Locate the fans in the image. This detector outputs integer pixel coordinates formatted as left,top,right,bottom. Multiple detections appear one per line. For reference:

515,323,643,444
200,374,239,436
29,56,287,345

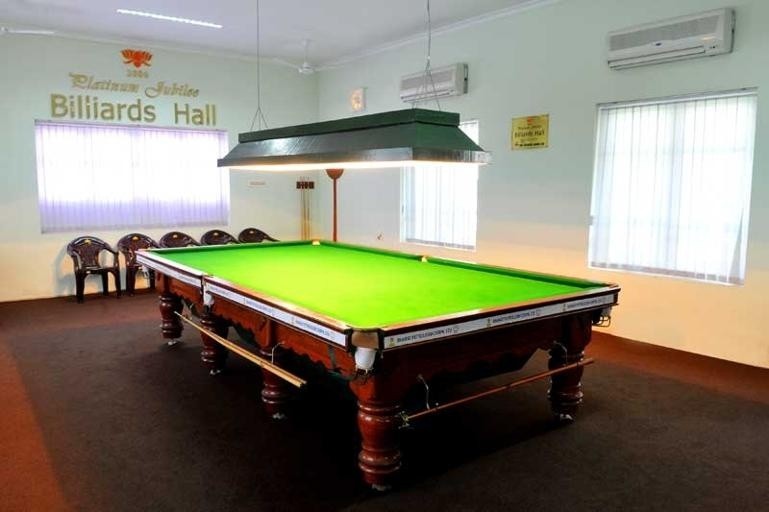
272,56,346,80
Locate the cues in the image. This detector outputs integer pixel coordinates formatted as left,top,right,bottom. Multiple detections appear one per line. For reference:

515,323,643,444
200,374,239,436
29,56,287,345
300,176,310,239
397,358,595,429
174,311,303,388
204,329,307,386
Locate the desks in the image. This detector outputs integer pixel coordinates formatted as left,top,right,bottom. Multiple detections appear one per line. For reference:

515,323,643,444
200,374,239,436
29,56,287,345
134,239,621,490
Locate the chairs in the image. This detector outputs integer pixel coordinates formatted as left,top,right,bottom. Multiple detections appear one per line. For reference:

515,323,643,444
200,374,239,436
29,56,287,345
238,228,281,243
159,231,204,248
201,229,241,246
67,236,121,304
118,232,163,296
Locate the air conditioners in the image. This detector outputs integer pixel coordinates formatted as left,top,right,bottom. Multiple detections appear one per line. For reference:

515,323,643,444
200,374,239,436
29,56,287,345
607,7,736,71
399,63,468,102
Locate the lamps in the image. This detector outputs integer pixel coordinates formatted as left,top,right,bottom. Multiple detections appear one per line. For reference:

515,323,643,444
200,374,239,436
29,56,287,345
217,108,493,170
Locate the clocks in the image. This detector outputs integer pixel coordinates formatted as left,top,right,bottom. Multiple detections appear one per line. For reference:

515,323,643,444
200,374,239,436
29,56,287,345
350,87,365,111
